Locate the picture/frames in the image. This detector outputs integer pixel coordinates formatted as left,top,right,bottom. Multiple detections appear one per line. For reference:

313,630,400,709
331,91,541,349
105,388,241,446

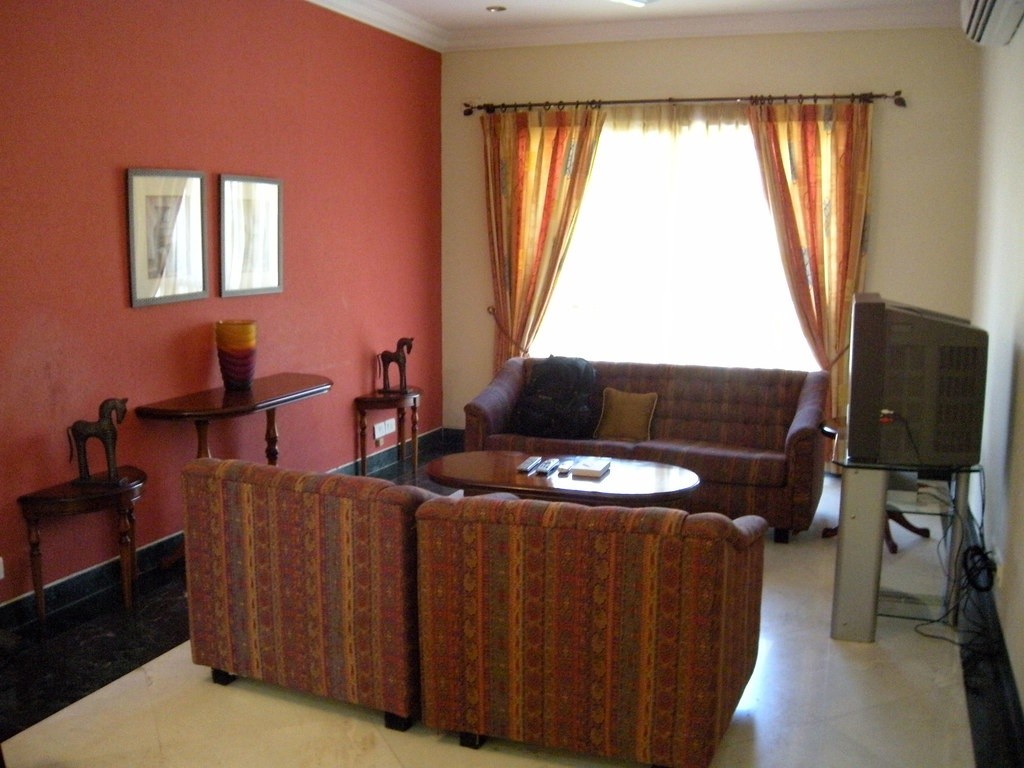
219,173,283,299
126,167,210,308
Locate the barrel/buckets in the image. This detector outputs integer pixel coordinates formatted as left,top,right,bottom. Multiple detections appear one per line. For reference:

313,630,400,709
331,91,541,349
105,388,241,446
215,319,256,393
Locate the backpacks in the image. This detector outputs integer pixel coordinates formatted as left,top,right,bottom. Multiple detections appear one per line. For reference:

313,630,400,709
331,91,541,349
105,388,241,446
513,356,598,440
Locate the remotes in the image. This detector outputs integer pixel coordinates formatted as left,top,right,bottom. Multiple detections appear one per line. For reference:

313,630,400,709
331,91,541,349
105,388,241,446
537,458,560,474
517,457,542,472
559,461,573,473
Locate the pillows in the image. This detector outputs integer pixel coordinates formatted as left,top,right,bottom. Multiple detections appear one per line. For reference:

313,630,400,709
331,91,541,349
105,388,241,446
592,388,658,443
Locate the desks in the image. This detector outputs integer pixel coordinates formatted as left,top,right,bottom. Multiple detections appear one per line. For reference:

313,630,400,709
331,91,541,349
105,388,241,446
425,450,700,511
135,373,333,468
830,430,983,645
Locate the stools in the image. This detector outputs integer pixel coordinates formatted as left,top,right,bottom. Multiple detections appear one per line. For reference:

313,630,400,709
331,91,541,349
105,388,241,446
820,416,930,554
16,465,148,628
353,385,425,478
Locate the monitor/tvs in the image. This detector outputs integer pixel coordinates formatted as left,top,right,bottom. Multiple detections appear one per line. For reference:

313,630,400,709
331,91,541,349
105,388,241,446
847,291,989,467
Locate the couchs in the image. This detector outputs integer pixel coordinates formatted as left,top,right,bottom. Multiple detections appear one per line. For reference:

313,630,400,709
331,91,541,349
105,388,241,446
416,493,768,768
181,457,464,732
464,354,830,545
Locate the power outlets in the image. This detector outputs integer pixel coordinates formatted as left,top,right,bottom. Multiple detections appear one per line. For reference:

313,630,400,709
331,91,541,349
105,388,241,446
992,547,1004,587
374,421,385,439
384,418,396,434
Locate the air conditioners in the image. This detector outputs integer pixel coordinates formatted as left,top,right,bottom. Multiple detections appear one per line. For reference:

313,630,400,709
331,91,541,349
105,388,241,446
960,0,1024,47
